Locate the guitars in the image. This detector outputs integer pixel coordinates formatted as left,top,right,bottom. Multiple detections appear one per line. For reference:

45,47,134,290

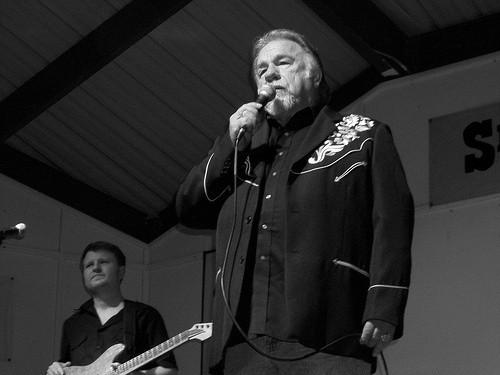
45,321,214,375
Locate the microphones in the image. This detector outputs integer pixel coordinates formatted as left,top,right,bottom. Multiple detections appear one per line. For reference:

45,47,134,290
0,223,26,241
237,84,276,141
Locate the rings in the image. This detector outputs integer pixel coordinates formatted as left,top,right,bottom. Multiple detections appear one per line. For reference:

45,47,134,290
241,109,247,115
381,333,391,343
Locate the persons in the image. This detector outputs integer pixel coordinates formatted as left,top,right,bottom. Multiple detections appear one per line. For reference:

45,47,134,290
176,27,413,375
45,240,179,375
